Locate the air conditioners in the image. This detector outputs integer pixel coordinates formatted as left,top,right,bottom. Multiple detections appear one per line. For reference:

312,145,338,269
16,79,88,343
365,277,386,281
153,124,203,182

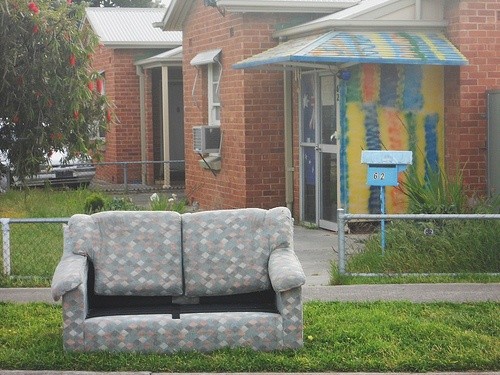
193,125,220,152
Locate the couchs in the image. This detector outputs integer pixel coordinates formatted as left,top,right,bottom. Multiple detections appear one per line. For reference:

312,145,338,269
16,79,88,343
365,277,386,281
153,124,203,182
51,207,306,354
